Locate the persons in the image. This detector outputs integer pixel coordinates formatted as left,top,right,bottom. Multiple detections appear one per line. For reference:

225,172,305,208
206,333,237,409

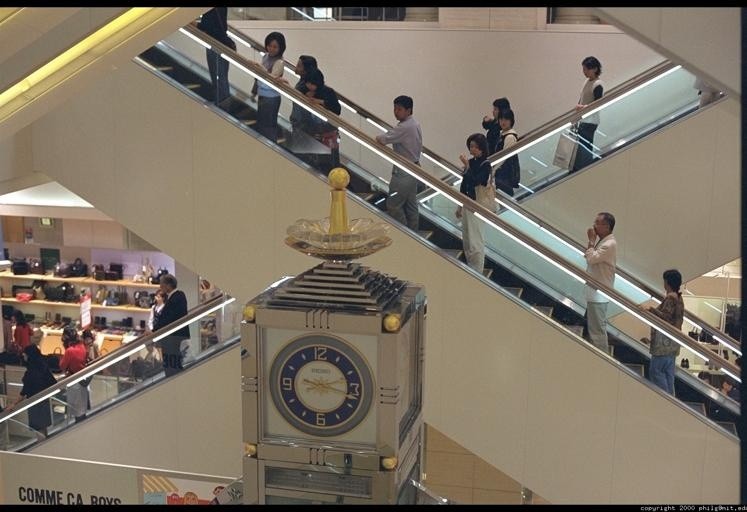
56,327,91,423
248,31,288,140
80,328,103,362
373,94,423,232
303,67,341,178
582,212,618,357
484,97,510,154
568,56,605,172
189,5,236,104
694,76,721,109
495,110,520,209
697,371,742,421
279,54,318,167
10,309,42,357
638,267,686,399
144,343,162,363
455,131,494,277
153,273,191,378
147,287,167,331
4,343,57,443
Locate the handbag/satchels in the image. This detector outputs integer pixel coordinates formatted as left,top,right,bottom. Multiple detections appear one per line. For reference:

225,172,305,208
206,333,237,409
553,133,579,173
224,35,237,50
417,163,426,195
476,182,500,212
322,129,339,150
0,258,217,419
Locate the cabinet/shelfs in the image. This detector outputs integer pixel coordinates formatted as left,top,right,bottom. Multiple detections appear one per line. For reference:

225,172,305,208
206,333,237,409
1,272,161,342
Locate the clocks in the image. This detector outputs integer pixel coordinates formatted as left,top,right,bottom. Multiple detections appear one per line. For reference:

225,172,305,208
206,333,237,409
268,334,373,435
